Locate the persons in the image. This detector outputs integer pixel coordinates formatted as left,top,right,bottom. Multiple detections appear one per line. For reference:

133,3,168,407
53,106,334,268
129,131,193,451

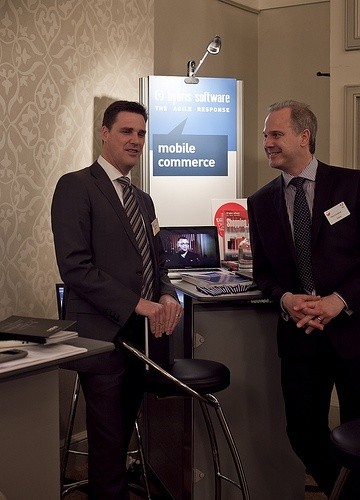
247,100,360,500
50,101,184,500
173,237,200,264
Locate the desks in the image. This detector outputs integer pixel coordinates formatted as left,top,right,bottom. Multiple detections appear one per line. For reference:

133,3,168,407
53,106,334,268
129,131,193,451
0,338,114,500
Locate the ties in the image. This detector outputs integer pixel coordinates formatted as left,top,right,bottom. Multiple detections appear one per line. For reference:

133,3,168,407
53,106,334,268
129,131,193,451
290,177,316,294
117,175,154,300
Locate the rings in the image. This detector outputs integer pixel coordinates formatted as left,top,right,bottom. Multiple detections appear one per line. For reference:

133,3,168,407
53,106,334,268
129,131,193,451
317,317,321,321
176,314,179,317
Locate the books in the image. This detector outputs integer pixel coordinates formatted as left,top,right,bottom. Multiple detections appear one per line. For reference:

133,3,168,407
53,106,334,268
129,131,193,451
0,315,78,344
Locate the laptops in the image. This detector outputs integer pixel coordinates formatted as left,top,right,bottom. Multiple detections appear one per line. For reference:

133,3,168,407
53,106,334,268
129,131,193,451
158,225,234,279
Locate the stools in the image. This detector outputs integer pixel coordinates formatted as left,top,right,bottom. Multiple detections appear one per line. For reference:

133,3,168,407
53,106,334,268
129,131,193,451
59,367,151,500
124,318,252,500
328,419,360,500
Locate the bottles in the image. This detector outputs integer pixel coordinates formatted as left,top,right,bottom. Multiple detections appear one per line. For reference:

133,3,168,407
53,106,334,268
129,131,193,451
238,226,253,272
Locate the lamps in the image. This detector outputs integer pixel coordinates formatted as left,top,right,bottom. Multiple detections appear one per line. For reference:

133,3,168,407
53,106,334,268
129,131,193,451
186,35,221,83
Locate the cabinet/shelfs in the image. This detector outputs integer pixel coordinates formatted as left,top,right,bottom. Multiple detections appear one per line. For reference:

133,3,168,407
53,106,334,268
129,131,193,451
170,274,307,500
